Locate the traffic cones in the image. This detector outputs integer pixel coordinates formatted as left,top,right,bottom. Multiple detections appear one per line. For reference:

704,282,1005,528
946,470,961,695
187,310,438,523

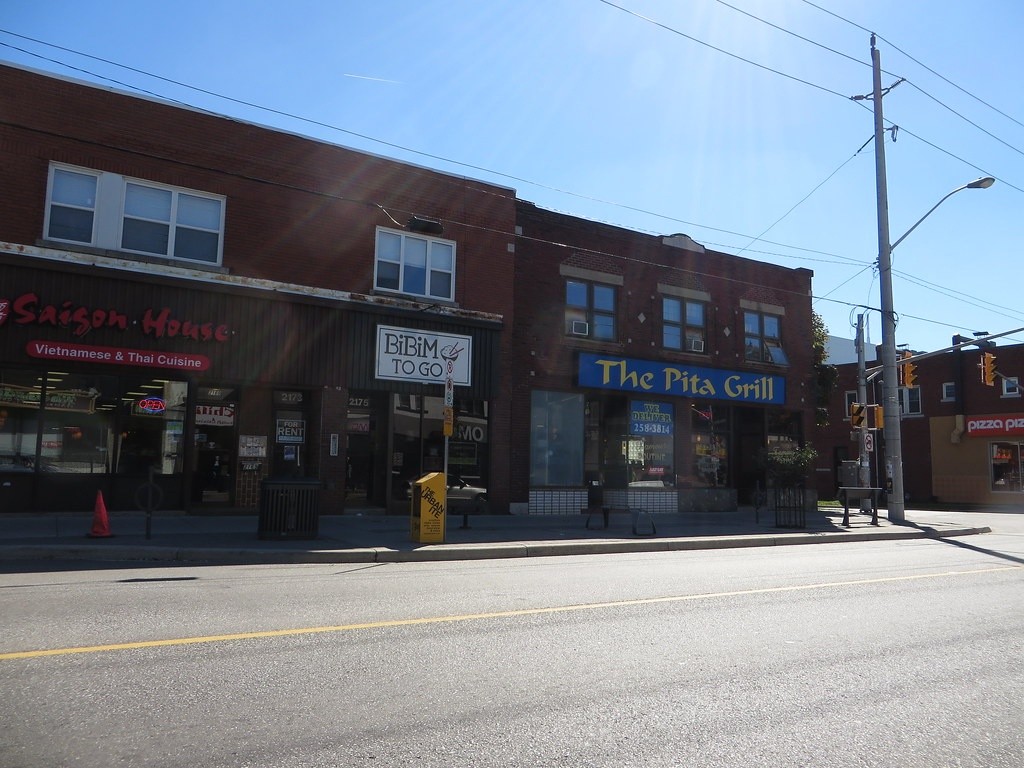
84,489,116,539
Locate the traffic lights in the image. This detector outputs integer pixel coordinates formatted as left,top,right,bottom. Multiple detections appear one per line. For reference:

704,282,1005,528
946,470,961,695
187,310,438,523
850,401,866,428
867,405,885,430
984,353,997,387
905,350,918,388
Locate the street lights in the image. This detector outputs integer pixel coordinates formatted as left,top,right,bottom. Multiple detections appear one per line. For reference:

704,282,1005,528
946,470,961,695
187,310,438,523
873,173,995,526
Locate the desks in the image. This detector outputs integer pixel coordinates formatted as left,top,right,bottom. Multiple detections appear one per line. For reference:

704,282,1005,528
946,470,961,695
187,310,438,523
584,504,658,538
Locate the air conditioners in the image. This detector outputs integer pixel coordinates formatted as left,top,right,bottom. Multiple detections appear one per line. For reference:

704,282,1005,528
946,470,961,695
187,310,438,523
570,320,590,337
686,339,705,353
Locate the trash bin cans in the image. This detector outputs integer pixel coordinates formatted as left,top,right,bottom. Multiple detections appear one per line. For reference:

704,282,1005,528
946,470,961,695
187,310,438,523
257,470,323,539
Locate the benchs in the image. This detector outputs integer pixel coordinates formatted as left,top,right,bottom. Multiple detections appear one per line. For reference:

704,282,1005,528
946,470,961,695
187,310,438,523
838,486,882,529
